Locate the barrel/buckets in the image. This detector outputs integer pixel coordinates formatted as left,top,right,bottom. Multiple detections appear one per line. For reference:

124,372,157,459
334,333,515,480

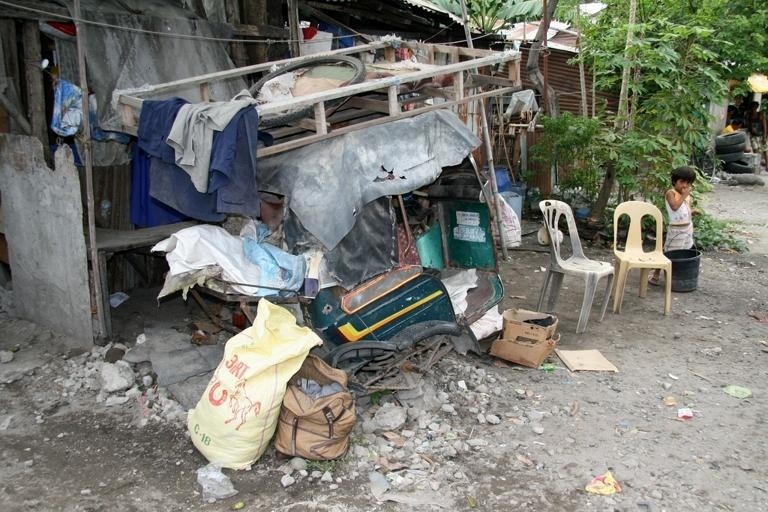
664,232,702,294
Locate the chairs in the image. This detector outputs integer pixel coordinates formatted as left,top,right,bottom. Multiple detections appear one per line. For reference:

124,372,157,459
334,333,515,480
537,199,615,335
611,200,673,317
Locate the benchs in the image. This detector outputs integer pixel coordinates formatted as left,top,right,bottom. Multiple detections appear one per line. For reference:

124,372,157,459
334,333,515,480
91,220,224,340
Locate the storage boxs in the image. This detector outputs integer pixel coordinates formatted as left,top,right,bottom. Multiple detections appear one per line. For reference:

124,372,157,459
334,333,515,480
490,308,562,369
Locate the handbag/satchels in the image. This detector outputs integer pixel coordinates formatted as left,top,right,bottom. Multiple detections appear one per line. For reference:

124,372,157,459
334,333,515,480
272,353,357,462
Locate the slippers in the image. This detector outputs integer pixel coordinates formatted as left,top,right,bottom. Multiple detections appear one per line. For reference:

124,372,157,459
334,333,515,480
649,274,661,285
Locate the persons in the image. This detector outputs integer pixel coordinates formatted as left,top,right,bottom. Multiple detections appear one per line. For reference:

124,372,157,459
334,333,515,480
721,94,768,171
649,165,704,286
